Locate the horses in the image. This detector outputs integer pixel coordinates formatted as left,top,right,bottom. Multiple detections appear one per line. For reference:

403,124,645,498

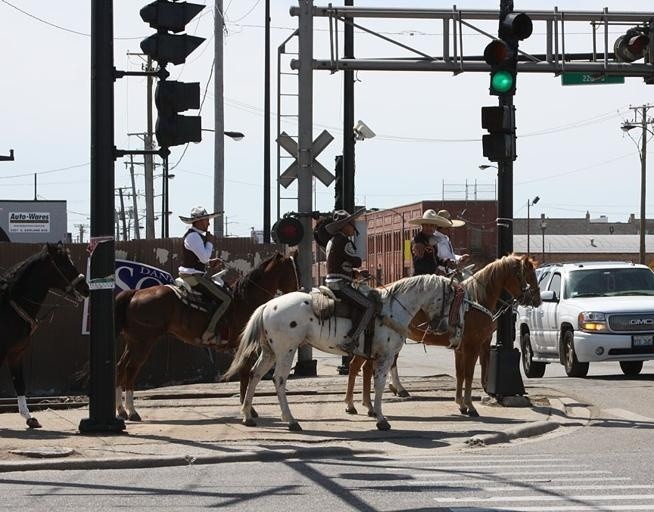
221,273,466,432
1,238,93,430
345,252,542,418
114,249,298,424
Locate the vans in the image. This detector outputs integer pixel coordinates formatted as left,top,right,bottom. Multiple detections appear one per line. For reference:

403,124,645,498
514,261,654,380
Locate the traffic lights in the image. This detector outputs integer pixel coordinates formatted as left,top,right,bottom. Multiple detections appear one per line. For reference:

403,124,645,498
140,0,209,67
481,13,533,98
478,105,518,161
149,78,204,148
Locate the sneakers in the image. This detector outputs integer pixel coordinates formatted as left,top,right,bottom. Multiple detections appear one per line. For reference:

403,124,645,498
432,325,448,336
342,337,360,355
204,335,228,346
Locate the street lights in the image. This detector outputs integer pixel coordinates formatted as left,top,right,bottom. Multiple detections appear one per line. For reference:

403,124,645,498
109,112,246,241
371,202,407,279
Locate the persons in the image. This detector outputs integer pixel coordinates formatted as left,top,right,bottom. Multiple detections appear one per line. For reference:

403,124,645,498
407,206,465,335
433,209,469,277
321,208,375,355
176,206,232,345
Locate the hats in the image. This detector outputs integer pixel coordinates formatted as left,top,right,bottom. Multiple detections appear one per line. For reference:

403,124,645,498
180,206,225,223
409,209,465,228
325,207,366,235
